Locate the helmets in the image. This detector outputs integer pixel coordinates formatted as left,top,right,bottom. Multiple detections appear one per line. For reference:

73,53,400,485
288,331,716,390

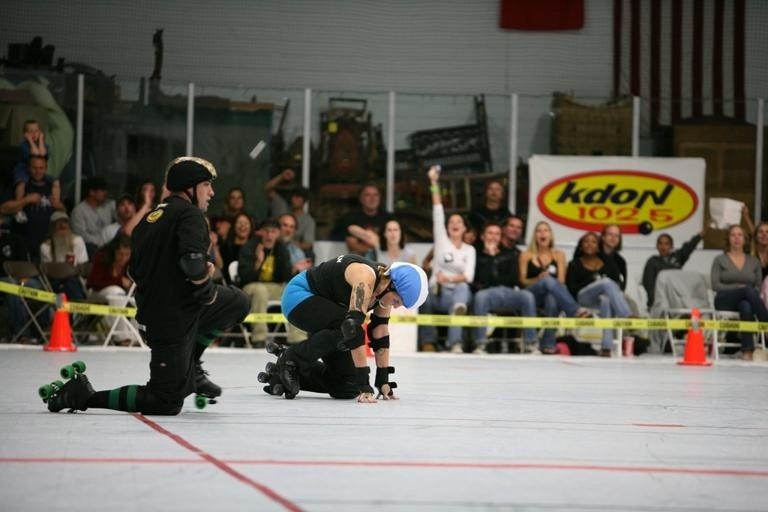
164,156,215,191
387,261,429,309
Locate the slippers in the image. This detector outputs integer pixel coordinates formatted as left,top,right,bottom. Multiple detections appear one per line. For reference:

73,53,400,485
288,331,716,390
540,344,561,354
571,310,594,319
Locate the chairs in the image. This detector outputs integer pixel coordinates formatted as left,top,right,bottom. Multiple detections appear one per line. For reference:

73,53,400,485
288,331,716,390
0,260,79,345
102,282,144,347
711,290,764,358
632,272,651,339
228,261,292,349
658,270,718,359
37,261,108,345
567,306,622,357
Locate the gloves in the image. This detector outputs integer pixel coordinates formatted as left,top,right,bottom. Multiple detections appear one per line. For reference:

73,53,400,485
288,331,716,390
374,367,399,397
352,367,375,394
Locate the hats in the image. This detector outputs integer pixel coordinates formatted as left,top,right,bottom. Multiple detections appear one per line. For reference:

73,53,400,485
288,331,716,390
116,192,135,204
86,176,107,188
49,209,69,223
258,216,283,230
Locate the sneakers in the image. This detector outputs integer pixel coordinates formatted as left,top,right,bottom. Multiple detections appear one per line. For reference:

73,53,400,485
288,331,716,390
266,343,312,400
451,341,464,354
628,316,648,333
258,362,284,397
14,208,29,225
597,346,614,355
190,362,221,410
452,301,468,316
525,343,544,356
38,360,96,415
472,344,487,353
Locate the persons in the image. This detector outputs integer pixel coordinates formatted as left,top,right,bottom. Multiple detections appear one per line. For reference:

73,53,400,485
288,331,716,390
518,222,600,356
599,225,652,356
113,190,158,238
712,225,768,362
278,213,308,273
136,180,159,215
420,165,478,354
212,217,235,247
239,217,292,283
643,222,717,342
346,186,395,252
209,215,256,287
567,233,649,358
263,168,316,250
744,202,768,314
86,239,134,346
472,224,541,355
464,181,515,243
0,154,67,261
50,157,252,417
8,120,66,223
499,216,525,286
222,187,248,218
70,172,122,248
378,219,418,274
32,210,88,350
276,253,428,404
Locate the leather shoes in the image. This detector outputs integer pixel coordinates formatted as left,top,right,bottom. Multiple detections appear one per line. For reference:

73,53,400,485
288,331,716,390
132,339,142,347
420,342,435,354
114,339,130,347
252,339,266,348
20,335,31,344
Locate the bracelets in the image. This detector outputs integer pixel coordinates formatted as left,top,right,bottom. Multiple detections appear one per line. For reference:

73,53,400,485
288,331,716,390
430,186,439,192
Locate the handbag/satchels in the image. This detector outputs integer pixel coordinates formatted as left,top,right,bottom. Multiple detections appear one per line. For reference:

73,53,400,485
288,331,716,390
42,236,79,281
